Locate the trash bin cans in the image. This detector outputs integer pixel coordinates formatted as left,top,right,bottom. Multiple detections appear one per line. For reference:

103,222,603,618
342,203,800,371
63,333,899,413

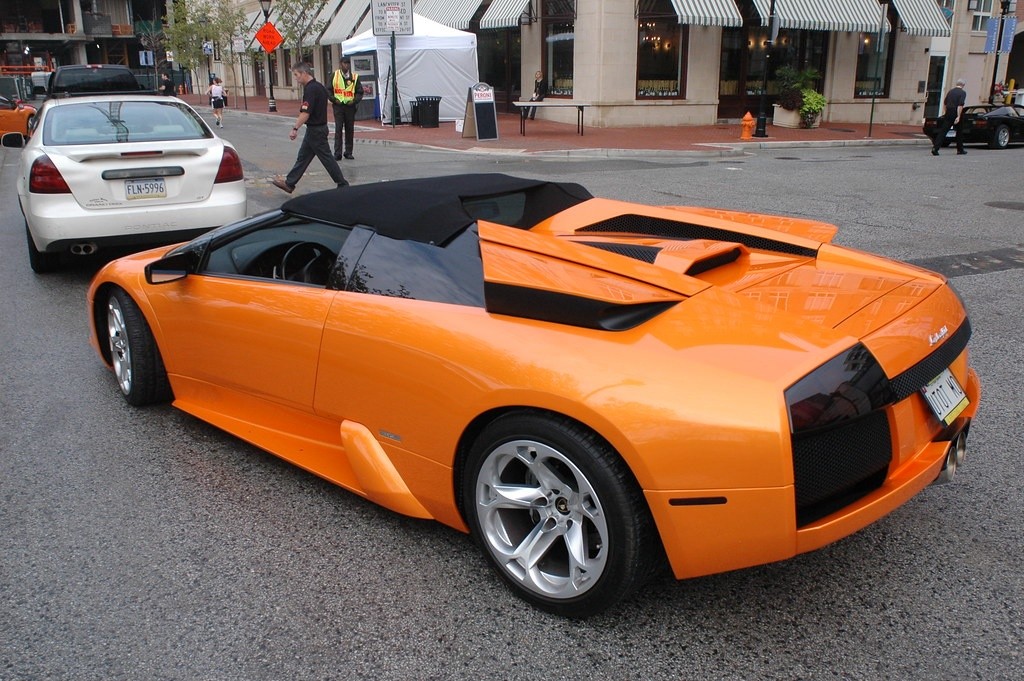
415,95,443,128
408,100,419,126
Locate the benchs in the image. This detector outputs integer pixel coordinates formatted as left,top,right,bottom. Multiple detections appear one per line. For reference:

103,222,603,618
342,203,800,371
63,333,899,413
66,124,184,136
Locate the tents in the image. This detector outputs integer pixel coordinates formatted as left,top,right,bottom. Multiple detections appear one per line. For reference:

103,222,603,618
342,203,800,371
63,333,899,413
340,11,479,125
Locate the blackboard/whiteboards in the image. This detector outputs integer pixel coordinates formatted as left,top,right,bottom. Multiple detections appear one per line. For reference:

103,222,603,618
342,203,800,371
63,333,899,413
461,83,499,141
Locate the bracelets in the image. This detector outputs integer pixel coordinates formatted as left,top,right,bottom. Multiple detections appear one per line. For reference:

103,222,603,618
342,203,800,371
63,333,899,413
293,128,298,130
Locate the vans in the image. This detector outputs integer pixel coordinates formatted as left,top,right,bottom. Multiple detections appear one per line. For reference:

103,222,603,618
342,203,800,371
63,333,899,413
33,64,143,96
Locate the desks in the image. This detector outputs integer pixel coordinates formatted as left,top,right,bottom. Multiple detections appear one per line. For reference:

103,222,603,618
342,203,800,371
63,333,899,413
513,102,591,135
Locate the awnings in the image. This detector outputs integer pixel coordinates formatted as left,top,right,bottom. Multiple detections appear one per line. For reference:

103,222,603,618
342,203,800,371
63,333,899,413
300,0,343,47
220,0,308,53
352,0,415,38
671,0,743,27
479,0,539,29
318,0,370,46
413,0,483,29
893,0,951,37
282,0,324,49
753,0,892,34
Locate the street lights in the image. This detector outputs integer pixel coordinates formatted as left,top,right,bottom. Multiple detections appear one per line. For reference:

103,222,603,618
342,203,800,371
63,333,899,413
987,0,1013,103
198,18,213,105
258,0,278,112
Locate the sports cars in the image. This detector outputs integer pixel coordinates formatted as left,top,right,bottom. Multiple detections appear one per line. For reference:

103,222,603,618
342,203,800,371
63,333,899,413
83,171,982,622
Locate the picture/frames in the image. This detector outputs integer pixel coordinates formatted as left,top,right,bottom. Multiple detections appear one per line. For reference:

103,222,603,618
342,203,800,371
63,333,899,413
360,80,376,99
350,55,374,76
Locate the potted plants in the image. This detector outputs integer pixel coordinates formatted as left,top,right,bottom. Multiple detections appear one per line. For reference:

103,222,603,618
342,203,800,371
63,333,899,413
771,64,827,129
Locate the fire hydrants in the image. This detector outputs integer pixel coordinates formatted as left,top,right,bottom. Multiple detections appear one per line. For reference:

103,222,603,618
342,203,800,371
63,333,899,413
739,111,757,141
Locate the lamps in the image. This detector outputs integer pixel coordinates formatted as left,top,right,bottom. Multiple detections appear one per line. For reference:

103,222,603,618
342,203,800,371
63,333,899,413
967,0,980,15
521,12,529,24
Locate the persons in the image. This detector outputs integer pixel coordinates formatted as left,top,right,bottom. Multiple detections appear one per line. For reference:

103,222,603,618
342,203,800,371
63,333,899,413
930,79,968,156
205,77,226,128
326,56,364,161
522,71,546,120
271,62,348,193
159,71,176,96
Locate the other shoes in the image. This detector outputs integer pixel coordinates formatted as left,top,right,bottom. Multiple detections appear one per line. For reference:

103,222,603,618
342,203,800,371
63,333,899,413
529,116,534,120
343,153,354,159
216,117,220,126
957,150,967,155
273,179,294,194
931,150,939,156
220,124,223,127
333,155,342,161
522,115,527,119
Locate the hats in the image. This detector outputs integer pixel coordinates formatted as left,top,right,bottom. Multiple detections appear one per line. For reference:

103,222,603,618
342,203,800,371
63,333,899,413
341,57,350,62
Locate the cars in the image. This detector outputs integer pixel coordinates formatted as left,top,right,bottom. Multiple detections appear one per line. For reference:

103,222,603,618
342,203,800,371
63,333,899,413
922,103,1024,149
1,94,248,278
0,95,38,145
